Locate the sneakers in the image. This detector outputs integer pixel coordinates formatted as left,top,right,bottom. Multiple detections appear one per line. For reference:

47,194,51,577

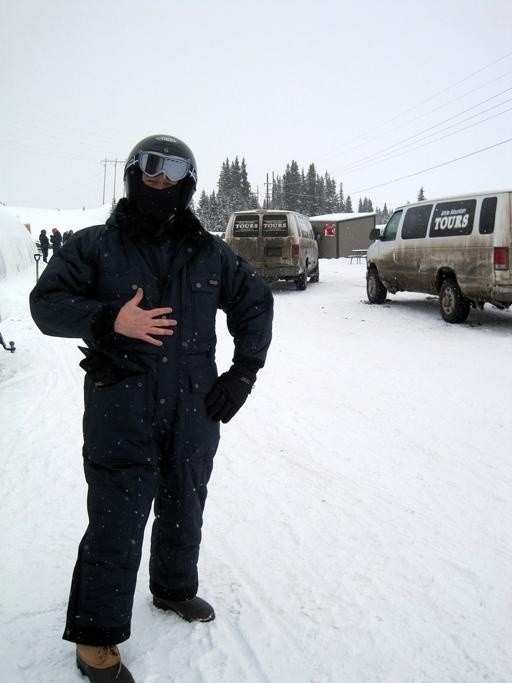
152,594,215,624
76,643,136,683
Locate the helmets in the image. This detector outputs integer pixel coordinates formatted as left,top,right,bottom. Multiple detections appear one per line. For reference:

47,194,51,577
124,134,197,177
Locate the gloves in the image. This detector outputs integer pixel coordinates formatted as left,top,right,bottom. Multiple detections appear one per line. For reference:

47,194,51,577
205,369,255,424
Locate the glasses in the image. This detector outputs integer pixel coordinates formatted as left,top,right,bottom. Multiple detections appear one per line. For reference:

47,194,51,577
128,150,197,183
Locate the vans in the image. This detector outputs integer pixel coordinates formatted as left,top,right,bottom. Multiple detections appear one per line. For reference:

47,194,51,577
366,190,511,324
222,209,321,290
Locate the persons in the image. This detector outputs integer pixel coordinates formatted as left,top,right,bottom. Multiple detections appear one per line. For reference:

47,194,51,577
39,230,49,263
30,135,274,683
63,230,73,244
50,228,62,255
